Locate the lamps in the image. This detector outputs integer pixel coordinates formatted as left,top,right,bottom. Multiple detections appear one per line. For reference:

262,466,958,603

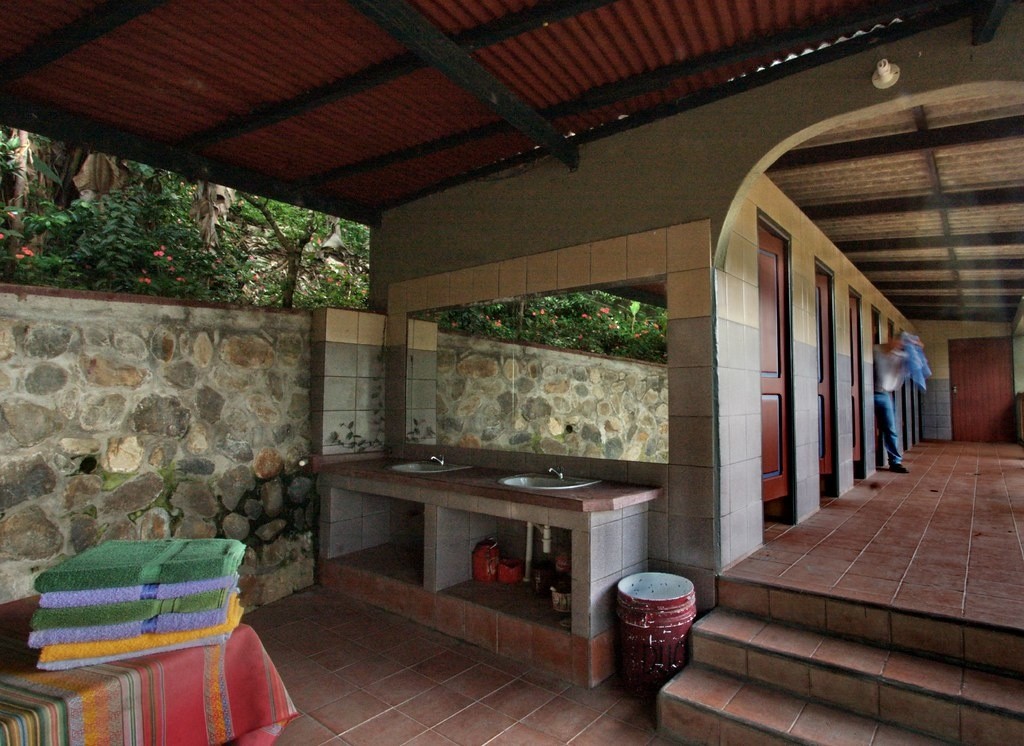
543,126,579,170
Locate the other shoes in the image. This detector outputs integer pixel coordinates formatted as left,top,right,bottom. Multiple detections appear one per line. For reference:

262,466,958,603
889,464,910,473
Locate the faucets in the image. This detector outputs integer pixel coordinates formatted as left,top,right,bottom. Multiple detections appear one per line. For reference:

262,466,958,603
429,453,445,467
547,468,564,480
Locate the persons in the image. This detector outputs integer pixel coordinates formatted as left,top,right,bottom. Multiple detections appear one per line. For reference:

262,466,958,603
872,332,924,472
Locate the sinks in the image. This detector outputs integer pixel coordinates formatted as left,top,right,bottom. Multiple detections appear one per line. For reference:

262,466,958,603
392,461,473,473
497,473,602,490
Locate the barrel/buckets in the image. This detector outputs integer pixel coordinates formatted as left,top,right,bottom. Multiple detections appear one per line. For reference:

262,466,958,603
616,572,696,696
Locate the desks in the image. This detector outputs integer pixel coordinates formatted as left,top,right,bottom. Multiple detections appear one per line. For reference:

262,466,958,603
0,595,298,746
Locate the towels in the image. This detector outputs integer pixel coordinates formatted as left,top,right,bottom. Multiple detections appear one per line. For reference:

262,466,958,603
898,330,931,391
27,539,248,670
